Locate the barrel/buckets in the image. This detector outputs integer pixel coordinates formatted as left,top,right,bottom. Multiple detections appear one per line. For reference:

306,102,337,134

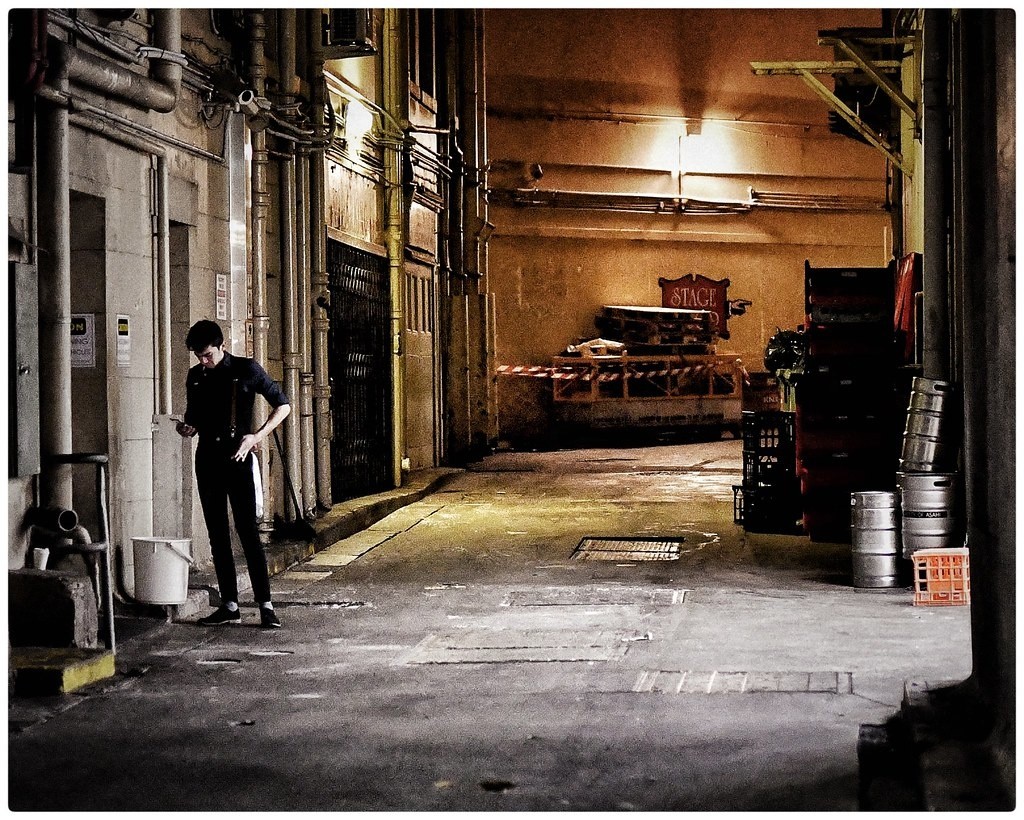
131,537,194,604
897,377,963,498
850,491,909,587
742,373,780,412
902,474,957,559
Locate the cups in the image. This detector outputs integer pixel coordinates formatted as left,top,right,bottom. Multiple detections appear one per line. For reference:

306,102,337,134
34,547,49,570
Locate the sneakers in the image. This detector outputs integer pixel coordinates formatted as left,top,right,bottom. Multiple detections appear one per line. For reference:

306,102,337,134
197,605,242,626
259,608,281,628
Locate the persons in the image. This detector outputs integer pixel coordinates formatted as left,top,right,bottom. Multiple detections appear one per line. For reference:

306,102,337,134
177,321,290,629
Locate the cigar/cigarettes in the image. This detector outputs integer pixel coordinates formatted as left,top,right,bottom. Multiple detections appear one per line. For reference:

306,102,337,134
239,453,243,459
